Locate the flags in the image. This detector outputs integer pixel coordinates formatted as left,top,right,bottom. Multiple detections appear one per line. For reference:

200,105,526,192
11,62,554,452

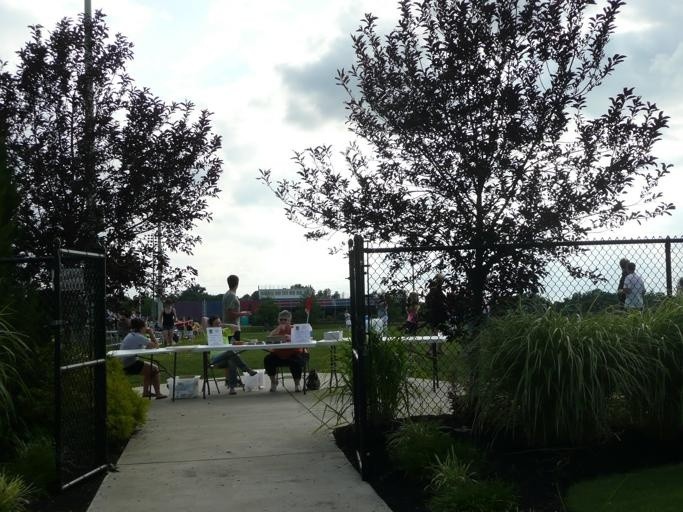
304,297,312,314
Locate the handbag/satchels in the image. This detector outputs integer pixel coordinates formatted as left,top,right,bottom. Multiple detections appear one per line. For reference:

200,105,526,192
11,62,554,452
305,368,320,390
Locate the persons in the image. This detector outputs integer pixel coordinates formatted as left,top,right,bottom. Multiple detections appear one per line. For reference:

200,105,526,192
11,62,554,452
344,311,352,336
118,317,168,400
676,278,683,297
622,263,646,313
405,291,421,336
263,309,308,393
158,299,199,355
222,274,253,388
617,257,630,309
365,314,369,331
375,293,389,338
204,314,258,395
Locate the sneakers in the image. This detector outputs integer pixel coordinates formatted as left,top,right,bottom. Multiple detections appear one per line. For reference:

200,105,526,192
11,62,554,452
295,384,301,392
156,394,167,399
270,381,278,392
143,393,155,397
250,371,257,376
229,392,237,395
